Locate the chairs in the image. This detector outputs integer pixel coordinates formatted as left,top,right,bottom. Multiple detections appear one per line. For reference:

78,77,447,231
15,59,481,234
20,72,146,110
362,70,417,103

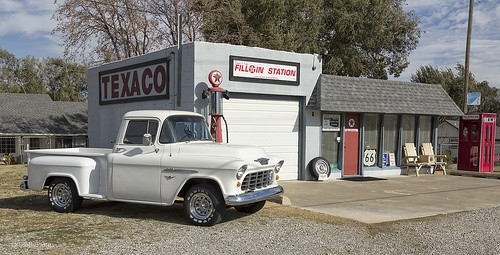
420,143,448,175
404,142,436,177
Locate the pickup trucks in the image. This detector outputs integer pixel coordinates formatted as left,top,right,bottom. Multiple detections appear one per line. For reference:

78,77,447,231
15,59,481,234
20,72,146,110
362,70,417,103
19,109,283,227
